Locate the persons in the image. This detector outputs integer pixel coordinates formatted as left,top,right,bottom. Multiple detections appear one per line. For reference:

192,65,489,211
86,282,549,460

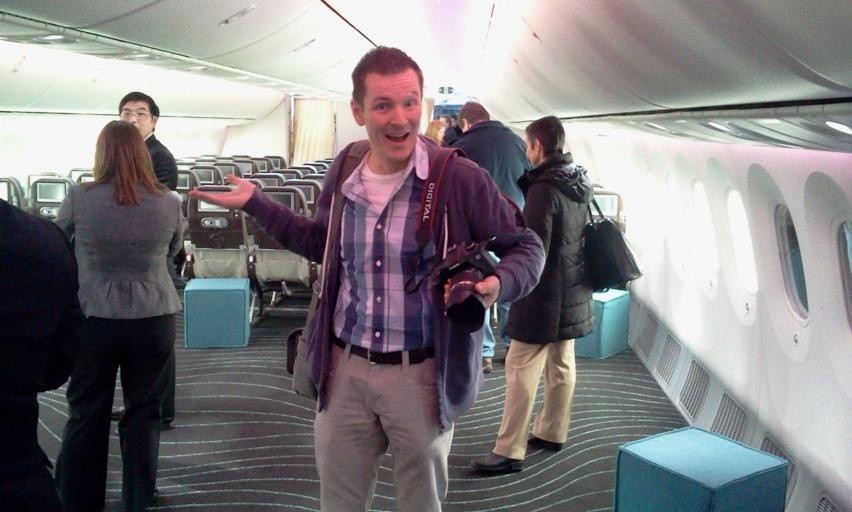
424,115,463,148
110,91,179,428
1,192,83,512
51,119,185,509
185,43,547,512
467,116,597,475
447,101,535,374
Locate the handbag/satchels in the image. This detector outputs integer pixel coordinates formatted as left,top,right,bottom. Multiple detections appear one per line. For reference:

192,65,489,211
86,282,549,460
582,220,648,294
282,329,319,401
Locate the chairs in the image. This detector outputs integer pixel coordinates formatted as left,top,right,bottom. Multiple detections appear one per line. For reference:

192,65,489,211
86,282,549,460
585,190,626,233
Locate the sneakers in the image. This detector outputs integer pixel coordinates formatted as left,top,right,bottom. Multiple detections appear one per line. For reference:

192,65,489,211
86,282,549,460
105,406,127,421
156,416,175,432
481,357,493,373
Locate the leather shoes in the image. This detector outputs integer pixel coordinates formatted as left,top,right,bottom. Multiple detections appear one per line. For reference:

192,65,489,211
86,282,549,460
526,433,563,453
469,449,525,475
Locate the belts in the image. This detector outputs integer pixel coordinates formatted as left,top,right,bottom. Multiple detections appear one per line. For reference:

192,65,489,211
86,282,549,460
331,332,434,367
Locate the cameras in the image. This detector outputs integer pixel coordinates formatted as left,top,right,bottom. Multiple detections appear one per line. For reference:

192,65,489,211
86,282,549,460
427,239,500,333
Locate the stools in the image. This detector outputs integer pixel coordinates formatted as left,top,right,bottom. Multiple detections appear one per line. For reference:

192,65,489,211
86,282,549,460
614,425,790,512
184,279,250,350
575,288,631,360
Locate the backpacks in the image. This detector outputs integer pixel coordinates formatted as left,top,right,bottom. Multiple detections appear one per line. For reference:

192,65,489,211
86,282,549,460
0,198,88,395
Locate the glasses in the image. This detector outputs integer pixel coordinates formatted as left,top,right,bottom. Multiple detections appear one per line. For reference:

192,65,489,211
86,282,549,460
121,110,153,122
